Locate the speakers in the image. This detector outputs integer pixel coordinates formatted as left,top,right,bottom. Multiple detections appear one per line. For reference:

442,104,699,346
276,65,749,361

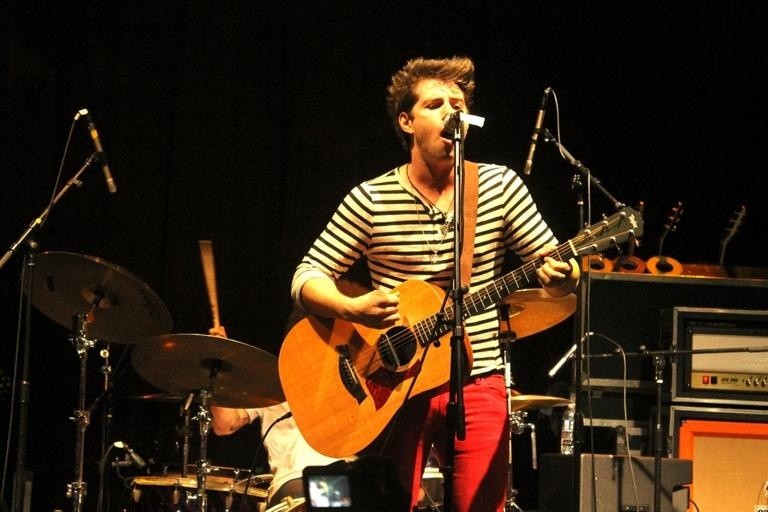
538,453,693,512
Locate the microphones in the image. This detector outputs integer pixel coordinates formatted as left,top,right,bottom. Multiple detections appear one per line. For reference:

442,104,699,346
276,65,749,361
445,110,465,135
522,90,549,175
548,331,594,379
86,115,117,194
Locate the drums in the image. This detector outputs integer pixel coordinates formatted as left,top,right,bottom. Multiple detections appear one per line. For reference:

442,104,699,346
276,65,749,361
132,474,270,512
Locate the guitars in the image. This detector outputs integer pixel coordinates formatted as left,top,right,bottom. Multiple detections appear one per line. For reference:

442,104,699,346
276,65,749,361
278,206,644,458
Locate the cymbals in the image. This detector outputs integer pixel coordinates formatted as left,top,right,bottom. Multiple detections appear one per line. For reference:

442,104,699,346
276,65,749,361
129,394,188,402
21,251,174,344
131,333,286,409
498,288,577,339
510,394,574,412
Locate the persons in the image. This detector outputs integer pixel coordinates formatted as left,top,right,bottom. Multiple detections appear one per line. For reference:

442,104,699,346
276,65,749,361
291,55,581,511
206,321,361,511
